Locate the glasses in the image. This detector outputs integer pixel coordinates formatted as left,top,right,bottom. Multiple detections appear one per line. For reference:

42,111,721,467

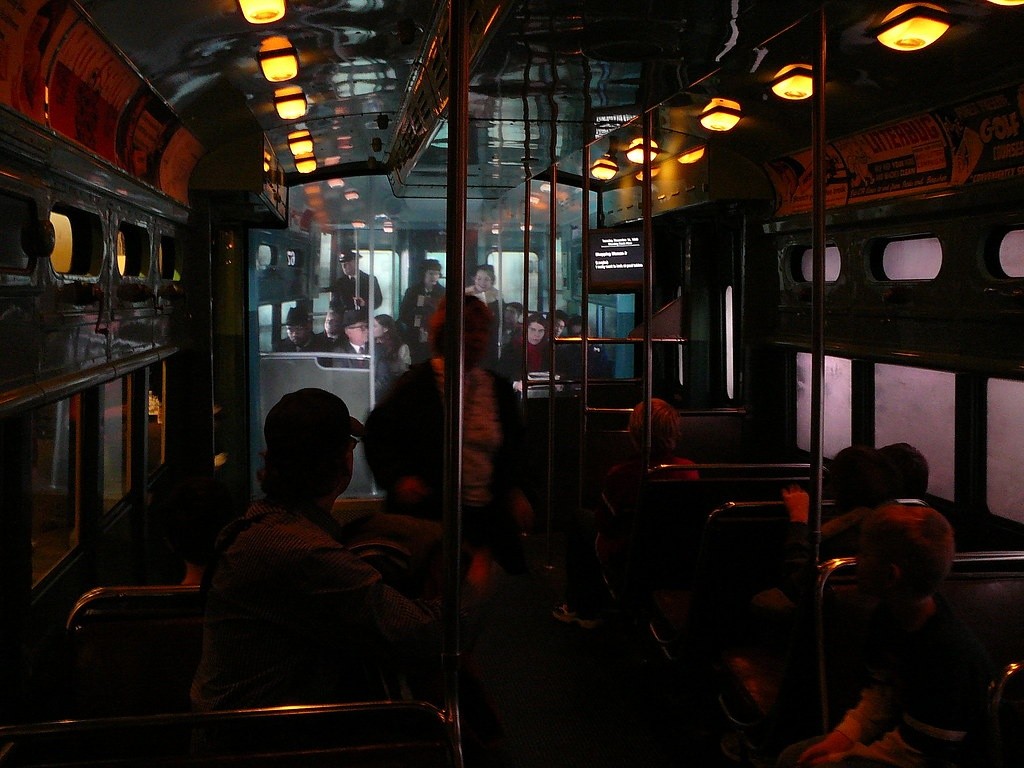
349,436,359,448
348,325,369,332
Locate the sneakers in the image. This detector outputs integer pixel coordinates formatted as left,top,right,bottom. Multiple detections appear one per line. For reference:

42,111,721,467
553,604,605,629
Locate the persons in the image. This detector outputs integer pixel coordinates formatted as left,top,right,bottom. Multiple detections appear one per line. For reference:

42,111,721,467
271,253,609,376
553,397,701,626
193,387,500,719
363,290,535,576
717,442,999,768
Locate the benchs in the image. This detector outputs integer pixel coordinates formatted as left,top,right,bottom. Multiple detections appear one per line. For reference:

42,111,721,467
0,536,465,768
601,463,1024,768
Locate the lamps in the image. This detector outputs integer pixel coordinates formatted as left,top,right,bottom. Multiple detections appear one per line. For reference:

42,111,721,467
257,36,300,82
274,85,307,120
768,67,814,100
625,138,660,164
288,130,314,154
864,1,961,51
237,0,287,24
697,97,744,131
590,158,620,180
295,158,318,174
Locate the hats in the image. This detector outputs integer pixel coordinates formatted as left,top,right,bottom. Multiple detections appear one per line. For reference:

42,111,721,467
264,388,367,443
336,310,367,327
279,307,315,327
339,252,361,263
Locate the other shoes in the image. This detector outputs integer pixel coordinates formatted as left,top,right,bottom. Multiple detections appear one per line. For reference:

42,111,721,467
720,734,769,767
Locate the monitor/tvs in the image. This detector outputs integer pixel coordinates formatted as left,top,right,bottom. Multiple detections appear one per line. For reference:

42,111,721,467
587,228,644,295
